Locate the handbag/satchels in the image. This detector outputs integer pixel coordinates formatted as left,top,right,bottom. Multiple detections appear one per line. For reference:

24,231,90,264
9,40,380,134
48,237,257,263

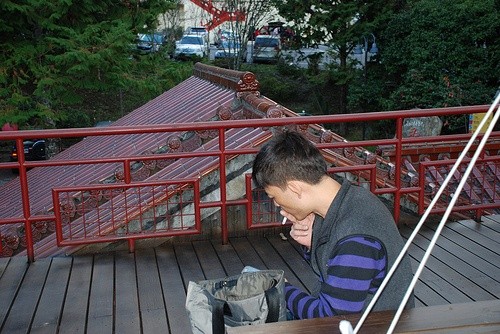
185,269,285,334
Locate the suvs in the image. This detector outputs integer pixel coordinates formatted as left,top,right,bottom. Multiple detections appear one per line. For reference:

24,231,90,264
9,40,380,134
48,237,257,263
175,35,208,61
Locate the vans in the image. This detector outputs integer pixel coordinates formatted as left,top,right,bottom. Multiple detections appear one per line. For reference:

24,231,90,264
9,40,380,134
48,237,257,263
137,32,173,59
251,35,283,64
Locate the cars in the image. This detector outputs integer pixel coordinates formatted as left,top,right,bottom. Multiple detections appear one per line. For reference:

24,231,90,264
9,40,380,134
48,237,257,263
214,29,240,49
214,40,249,63
184,26,209,40
0,127,63,174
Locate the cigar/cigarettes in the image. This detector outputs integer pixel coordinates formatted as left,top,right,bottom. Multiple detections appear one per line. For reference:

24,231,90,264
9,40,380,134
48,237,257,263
282,217,287,225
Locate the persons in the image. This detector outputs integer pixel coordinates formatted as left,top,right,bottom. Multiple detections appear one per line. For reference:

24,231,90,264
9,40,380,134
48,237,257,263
408,119,451,137
252,128,415,321
215,24,296,52
